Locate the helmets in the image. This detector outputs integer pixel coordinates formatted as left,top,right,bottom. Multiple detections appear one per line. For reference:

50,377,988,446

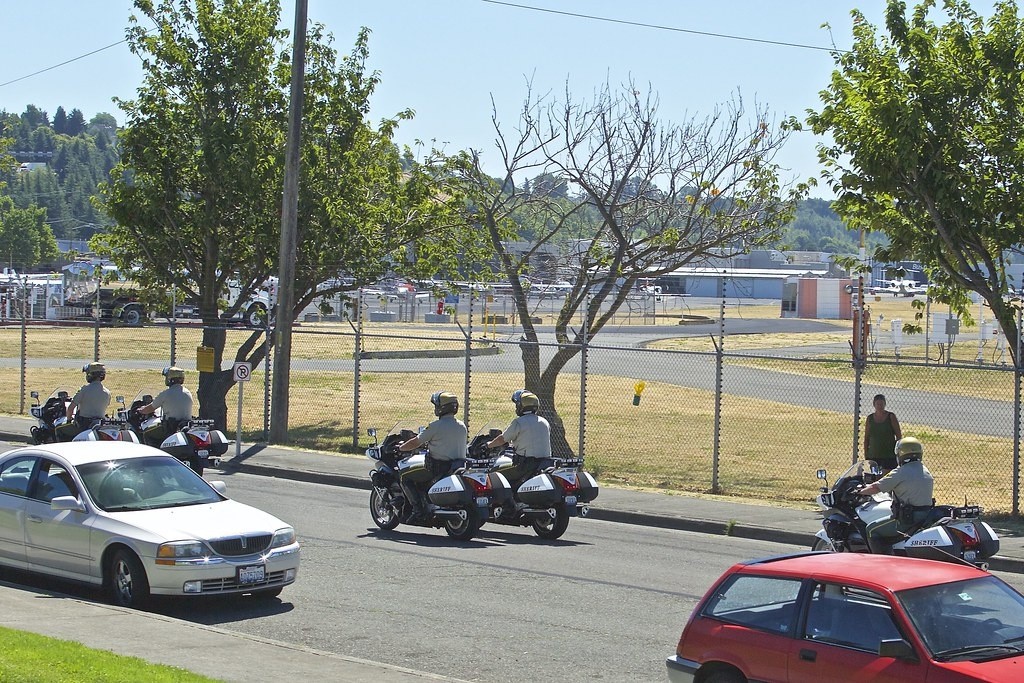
516,391,540,417
86,362,105,381
895,438,922,459
164,367,184,385
434,392,458,416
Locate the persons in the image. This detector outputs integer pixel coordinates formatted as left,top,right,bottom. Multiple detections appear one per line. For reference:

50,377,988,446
54,362,112,442
137,366,193,447
851,437,934,556
483,390,551,518
397,391,467,524
863,395,902,475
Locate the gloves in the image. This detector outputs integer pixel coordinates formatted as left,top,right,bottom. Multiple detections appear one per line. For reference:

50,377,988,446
475,444,489,454
390,444,399,453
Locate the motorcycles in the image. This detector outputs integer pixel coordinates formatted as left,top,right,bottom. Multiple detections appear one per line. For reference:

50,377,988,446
28,385,140,446
115,388,228,478
466,419,599,539
366,420,503,541
811,460,1000,572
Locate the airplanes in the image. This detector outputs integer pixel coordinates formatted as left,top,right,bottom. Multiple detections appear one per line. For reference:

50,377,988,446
852,266,928,297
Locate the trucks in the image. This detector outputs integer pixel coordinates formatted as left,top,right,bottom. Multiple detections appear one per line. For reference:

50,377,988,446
64,272,305,328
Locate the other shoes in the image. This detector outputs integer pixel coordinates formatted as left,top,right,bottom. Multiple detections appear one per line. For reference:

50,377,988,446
488,501,516,519
406,509,426,525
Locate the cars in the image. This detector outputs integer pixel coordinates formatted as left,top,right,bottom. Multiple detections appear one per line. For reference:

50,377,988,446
308,274,587,300
0,441,301,610
852,284,952,295
964,277,1024,295
666,551,1024,683
